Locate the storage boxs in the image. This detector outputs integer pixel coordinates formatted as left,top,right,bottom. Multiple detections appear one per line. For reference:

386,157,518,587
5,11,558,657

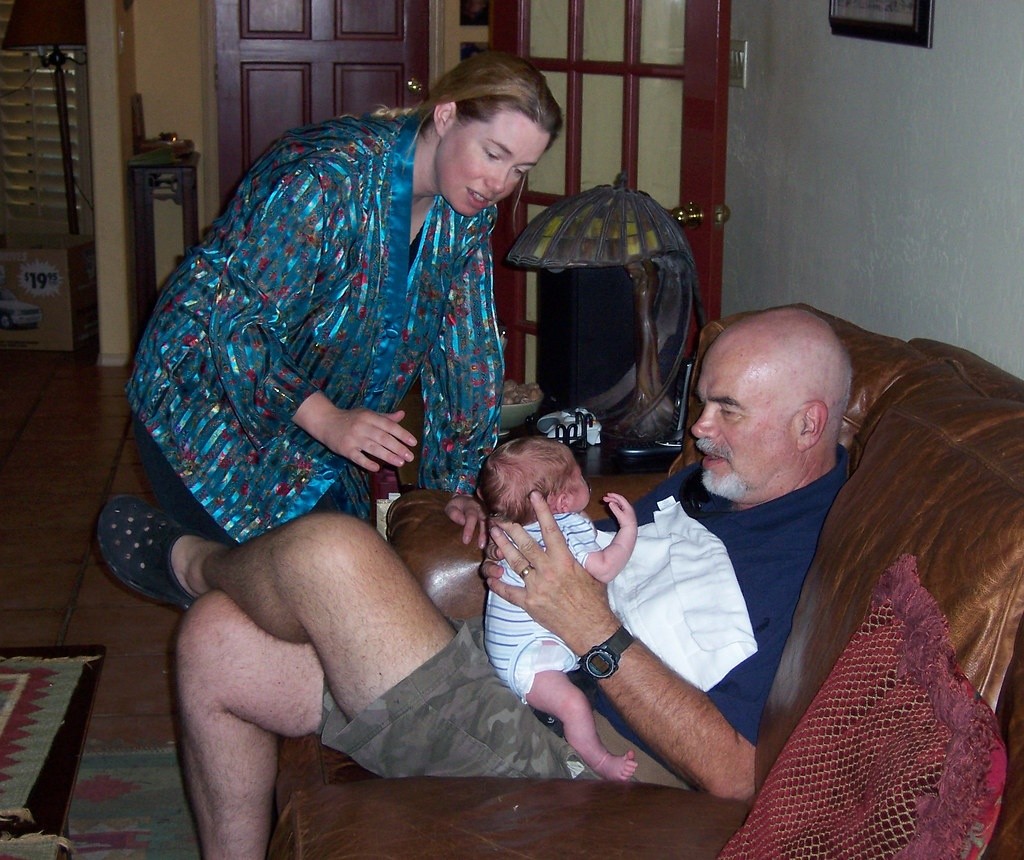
0,234,95,352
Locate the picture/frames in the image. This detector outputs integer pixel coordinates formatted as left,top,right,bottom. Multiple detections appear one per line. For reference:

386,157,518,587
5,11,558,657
828,0,935,48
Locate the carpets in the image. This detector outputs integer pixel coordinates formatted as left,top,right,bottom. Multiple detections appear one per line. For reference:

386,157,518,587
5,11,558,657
65,740,201,860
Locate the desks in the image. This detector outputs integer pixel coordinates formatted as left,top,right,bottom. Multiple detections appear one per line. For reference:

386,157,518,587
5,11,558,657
128,152,202,350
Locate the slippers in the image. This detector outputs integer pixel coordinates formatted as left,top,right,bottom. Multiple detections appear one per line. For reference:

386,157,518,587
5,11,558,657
96,494,199,609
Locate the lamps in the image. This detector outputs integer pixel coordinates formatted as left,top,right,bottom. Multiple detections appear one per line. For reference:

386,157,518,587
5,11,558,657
508,171,696,476
0,0,89,237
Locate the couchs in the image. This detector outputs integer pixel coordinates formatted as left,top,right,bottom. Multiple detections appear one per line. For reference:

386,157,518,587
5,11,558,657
271,302,1024,860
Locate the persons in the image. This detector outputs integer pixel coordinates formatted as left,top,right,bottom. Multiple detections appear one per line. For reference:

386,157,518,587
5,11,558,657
119,50,564,548
96,306,852,860
478,434,639,782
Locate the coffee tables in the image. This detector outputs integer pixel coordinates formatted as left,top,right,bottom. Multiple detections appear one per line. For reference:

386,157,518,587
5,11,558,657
0,641,107,860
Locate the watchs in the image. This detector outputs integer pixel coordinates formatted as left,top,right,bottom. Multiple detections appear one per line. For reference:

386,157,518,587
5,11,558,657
577,624,636,682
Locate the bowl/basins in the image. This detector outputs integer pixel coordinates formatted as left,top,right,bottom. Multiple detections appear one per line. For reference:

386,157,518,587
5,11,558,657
498,390,543,435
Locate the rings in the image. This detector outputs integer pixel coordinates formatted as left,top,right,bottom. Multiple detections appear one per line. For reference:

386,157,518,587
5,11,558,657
519,565,534,580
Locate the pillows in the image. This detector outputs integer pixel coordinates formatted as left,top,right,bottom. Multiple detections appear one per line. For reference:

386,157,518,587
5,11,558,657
714,556,1008,860
